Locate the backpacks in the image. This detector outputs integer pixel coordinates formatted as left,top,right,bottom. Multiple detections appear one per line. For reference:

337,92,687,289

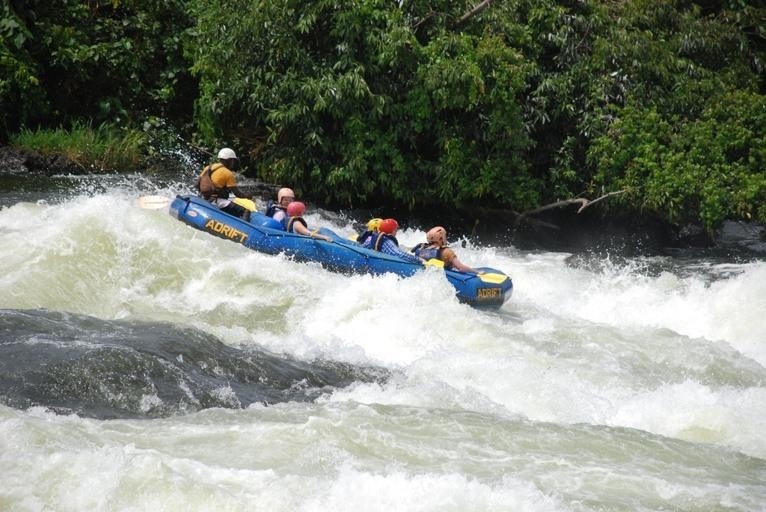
199,164,224,194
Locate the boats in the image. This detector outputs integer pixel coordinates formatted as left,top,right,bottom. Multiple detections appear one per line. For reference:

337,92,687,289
168,194,513,313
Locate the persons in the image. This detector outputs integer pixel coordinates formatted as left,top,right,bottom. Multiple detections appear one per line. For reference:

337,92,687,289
368,218,427,266
265,188,294,222
407,242,428,256
282,201,334,242
417,226,486,275
193,148,258,223
356,217,384,248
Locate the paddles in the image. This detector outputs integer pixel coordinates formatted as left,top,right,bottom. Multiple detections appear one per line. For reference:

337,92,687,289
231,196,258,213
470,272,508,285
421,258,445,270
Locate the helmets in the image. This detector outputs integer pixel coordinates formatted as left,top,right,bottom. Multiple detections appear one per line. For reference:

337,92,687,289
287,202,305,215
278,188,294,203
426,226,447,244
367,218,398,233
217,147,237,160
